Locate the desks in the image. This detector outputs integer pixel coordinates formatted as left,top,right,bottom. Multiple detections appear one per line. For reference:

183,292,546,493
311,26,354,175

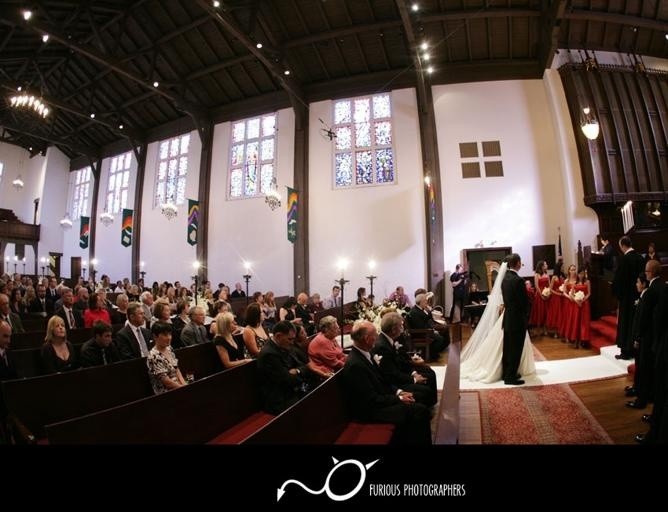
464,304,487,325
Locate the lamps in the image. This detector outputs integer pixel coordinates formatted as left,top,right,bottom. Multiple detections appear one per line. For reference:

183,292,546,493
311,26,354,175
10,82,51,123
263,177,282,211
12,146,27,193
564,43,599,140
157,202,178,221
100,205,114,225
61,212,74,230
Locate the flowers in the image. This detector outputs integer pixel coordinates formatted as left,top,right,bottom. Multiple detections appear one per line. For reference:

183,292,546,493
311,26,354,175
574,291,584,302
556,283,565,291
542,286,550,296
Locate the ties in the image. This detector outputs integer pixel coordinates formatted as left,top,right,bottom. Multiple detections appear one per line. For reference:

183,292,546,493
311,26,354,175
42,299,45,311
69,310,76,329
52,290,54,296
136,329,149,357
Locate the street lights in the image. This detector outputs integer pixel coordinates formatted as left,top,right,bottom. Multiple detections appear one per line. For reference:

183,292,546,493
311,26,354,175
5,255,98,286
241,260,252,305
138,260,148,289
191,257,201,306
365,257,378,309
335,255,350,350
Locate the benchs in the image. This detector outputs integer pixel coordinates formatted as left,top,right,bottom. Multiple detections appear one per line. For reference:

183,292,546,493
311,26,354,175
0,275,449,446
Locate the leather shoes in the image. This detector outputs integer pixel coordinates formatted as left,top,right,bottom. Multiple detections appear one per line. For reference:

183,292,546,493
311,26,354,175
641,415,652,423
635,434,646,442
501,374,525,384
625,388,636,397
625,400,647,409
